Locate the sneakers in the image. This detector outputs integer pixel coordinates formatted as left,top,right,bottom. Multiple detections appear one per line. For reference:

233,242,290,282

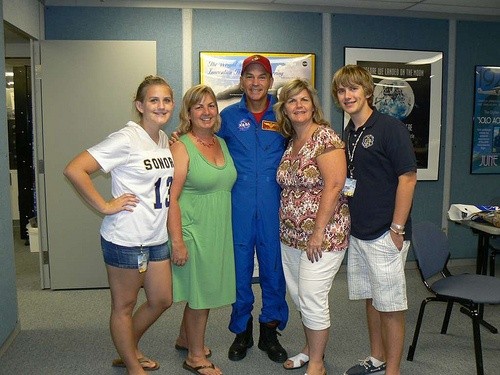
343,355,387,375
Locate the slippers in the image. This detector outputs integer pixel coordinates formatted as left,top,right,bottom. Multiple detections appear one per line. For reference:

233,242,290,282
112,356,160,371
175,343,223,375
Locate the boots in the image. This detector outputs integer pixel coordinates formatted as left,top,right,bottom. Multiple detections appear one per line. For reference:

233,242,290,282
257,319,288,363
228,314,254,361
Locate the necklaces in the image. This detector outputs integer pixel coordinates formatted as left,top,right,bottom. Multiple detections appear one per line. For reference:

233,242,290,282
190,131,215,148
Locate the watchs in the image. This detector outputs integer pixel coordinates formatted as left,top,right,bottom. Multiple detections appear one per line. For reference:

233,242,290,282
391,223,405,230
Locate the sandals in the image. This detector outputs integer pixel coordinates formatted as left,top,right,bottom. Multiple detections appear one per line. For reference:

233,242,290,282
283,352,328,375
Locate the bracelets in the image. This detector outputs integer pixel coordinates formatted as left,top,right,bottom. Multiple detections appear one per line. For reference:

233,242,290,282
390,227,405,234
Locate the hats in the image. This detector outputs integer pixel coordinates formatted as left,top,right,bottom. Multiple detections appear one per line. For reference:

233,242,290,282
241,55,272,78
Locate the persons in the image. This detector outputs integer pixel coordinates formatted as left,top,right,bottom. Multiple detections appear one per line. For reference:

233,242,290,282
169,56,290,362
276,79,351,375
167,84,237,375
332,66,417,375
64,76,175,375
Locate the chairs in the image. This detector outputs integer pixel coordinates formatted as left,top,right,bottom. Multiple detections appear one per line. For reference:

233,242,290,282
410,222,500,375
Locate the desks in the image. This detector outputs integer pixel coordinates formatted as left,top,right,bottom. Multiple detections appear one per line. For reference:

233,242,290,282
448,218,500,276
